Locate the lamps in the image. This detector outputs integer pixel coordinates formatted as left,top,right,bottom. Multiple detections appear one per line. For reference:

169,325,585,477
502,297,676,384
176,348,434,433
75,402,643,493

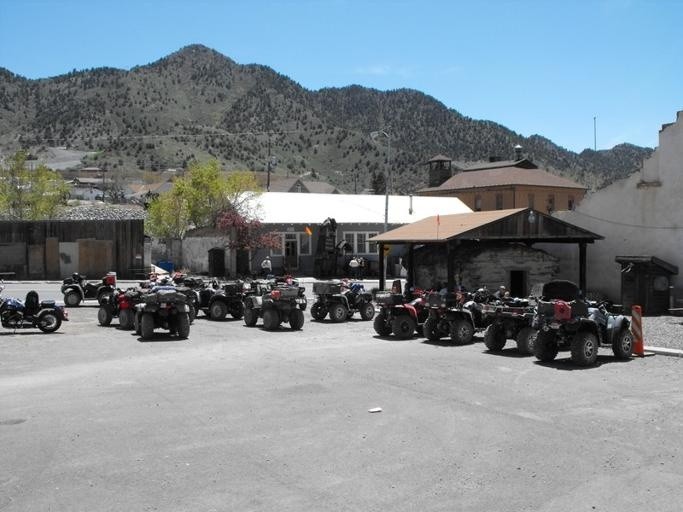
527,208,535,225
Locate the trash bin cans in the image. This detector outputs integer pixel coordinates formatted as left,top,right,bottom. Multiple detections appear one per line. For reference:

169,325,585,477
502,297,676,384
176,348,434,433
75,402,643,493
160,262,173,276
208,247,224,277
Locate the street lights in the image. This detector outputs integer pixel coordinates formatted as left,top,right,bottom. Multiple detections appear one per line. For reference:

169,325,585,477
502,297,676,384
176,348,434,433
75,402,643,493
369,129,390,281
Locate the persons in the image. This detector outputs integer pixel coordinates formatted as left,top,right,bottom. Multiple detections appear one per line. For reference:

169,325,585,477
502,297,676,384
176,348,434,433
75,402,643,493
261,256,272,279
349,256,365,280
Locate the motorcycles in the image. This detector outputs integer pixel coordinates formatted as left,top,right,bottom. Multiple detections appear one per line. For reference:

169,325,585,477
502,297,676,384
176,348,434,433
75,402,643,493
0,281,69,333
